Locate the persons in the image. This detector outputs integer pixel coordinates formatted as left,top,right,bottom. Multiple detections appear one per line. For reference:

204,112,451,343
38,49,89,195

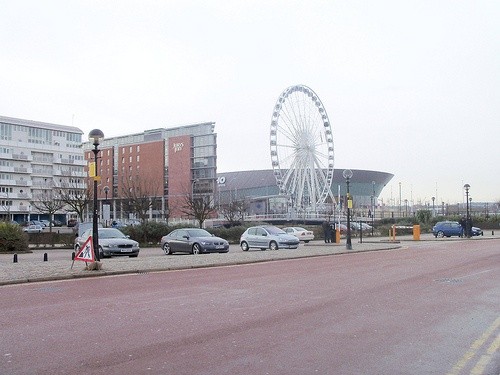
459,215,473,238
322,219,332,243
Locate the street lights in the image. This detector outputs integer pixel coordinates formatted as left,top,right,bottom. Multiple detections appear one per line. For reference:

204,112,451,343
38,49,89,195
88,129,105,262
442,201,459,216
379,182,430,218
432,196,435,209
343,169,353,249
371,180,376,218
464,183,471,238
469,198,472,213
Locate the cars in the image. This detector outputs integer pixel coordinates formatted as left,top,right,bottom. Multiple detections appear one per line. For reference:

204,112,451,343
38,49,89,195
22,219,62,228
344,222,366,231
282,226,314,243
240,225,300,252
75,227,140,260
67,219,77,227
335,223,352,233
360,222,374,229
432,221,481,238
160,228,229,255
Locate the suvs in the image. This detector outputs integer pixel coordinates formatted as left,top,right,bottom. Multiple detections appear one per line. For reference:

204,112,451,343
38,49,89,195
21,225,42,233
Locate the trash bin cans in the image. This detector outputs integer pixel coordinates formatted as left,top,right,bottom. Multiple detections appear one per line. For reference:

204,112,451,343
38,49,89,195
329,223,336,242
